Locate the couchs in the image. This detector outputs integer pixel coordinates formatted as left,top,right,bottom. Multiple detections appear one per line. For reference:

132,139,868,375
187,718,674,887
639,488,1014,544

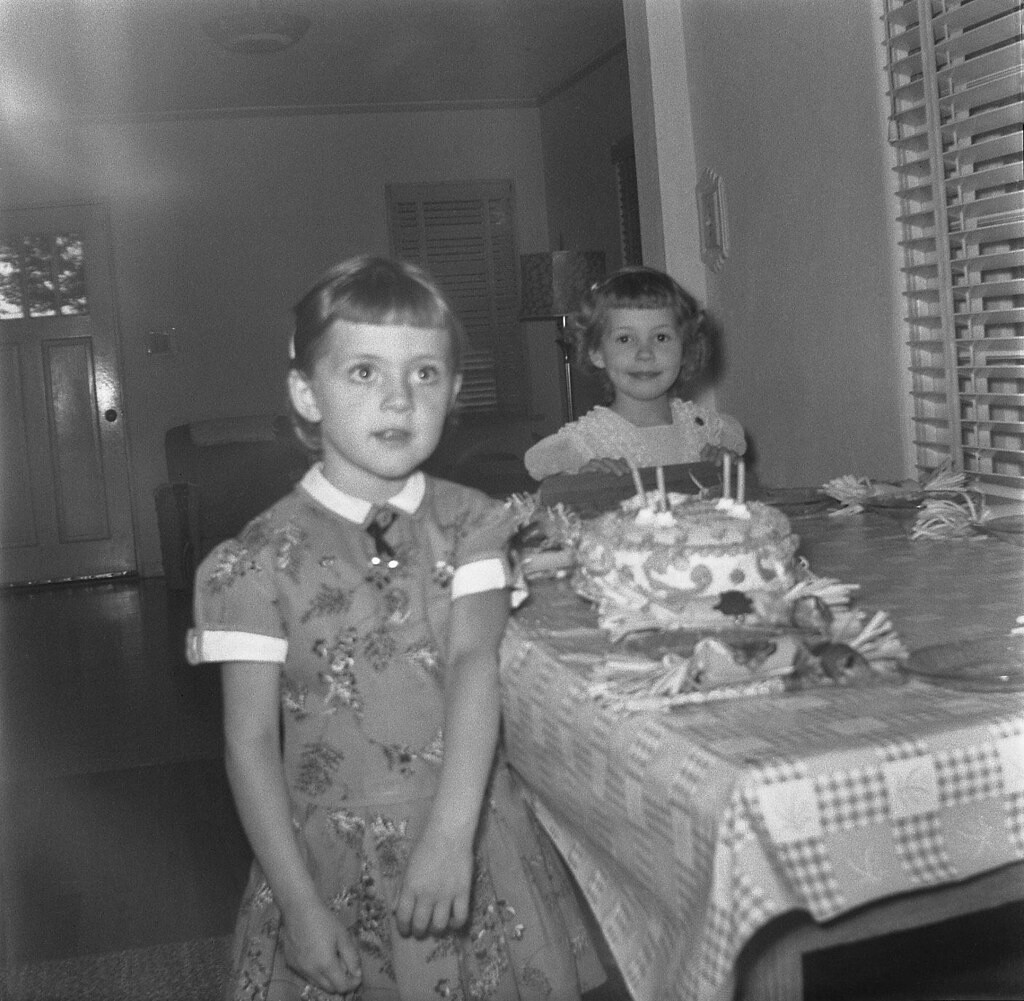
152,414,319,592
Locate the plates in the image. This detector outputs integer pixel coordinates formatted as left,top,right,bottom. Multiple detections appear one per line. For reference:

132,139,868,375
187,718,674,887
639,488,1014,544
900,635,1024,692
627,628,820,662
751,487,838,517
982,514,1024,547
862,489,978,518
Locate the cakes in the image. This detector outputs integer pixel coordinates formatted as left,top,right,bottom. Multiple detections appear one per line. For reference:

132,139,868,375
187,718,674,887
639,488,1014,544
573,495,798,602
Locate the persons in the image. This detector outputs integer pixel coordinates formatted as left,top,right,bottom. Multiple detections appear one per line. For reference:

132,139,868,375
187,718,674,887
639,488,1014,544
185,252,609,1000
523,265,748,478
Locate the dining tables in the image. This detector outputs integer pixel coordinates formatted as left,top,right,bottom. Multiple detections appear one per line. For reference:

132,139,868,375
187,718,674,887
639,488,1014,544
489,505,1023,1001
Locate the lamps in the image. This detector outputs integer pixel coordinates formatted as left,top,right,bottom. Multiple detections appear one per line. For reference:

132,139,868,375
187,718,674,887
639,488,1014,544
519,250,606,422
